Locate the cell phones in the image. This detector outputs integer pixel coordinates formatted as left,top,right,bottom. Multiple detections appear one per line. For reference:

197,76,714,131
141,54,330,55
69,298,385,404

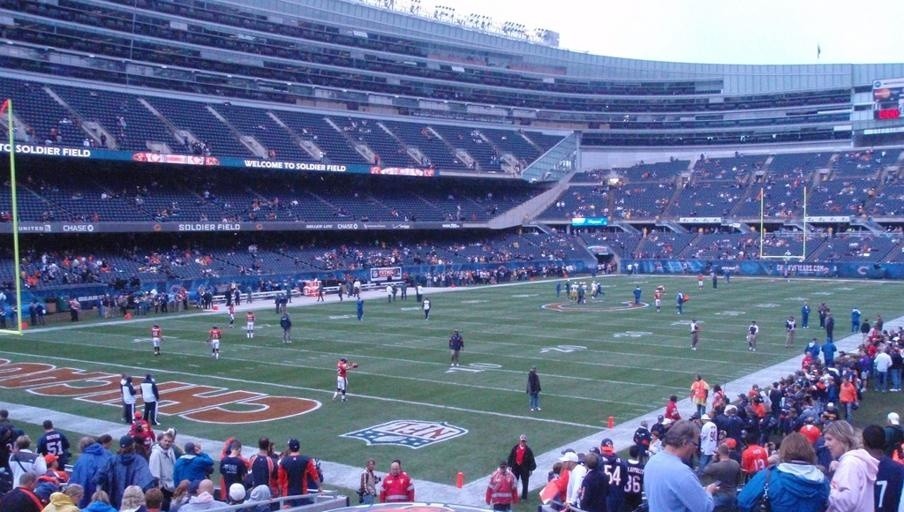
714,480,722,486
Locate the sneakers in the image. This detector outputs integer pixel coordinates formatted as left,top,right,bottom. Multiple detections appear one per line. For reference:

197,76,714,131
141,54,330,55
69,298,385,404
332,398,349,403
690,345,696,351
748,346,756,352
528,407,543,414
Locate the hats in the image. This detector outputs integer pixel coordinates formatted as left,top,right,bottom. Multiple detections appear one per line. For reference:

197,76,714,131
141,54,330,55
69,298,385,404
134,410,143,418
499,459,508,467
519,434,529,443
288,438,301,450
700,413,712,421
228,482,247,502
600,437,615,452
887,410,901,426
557,451,580,464
119,435,135,449
44,454,59,465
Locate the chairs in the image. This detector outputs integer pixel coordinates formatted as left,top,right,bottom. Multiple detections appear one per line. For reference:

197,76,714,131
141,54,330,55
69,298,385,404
0,0,903,295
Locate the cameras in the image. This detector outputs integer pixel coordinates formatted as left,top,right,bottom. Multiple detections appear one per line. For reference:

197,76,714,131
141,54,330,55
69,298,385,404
356,491,364,503
